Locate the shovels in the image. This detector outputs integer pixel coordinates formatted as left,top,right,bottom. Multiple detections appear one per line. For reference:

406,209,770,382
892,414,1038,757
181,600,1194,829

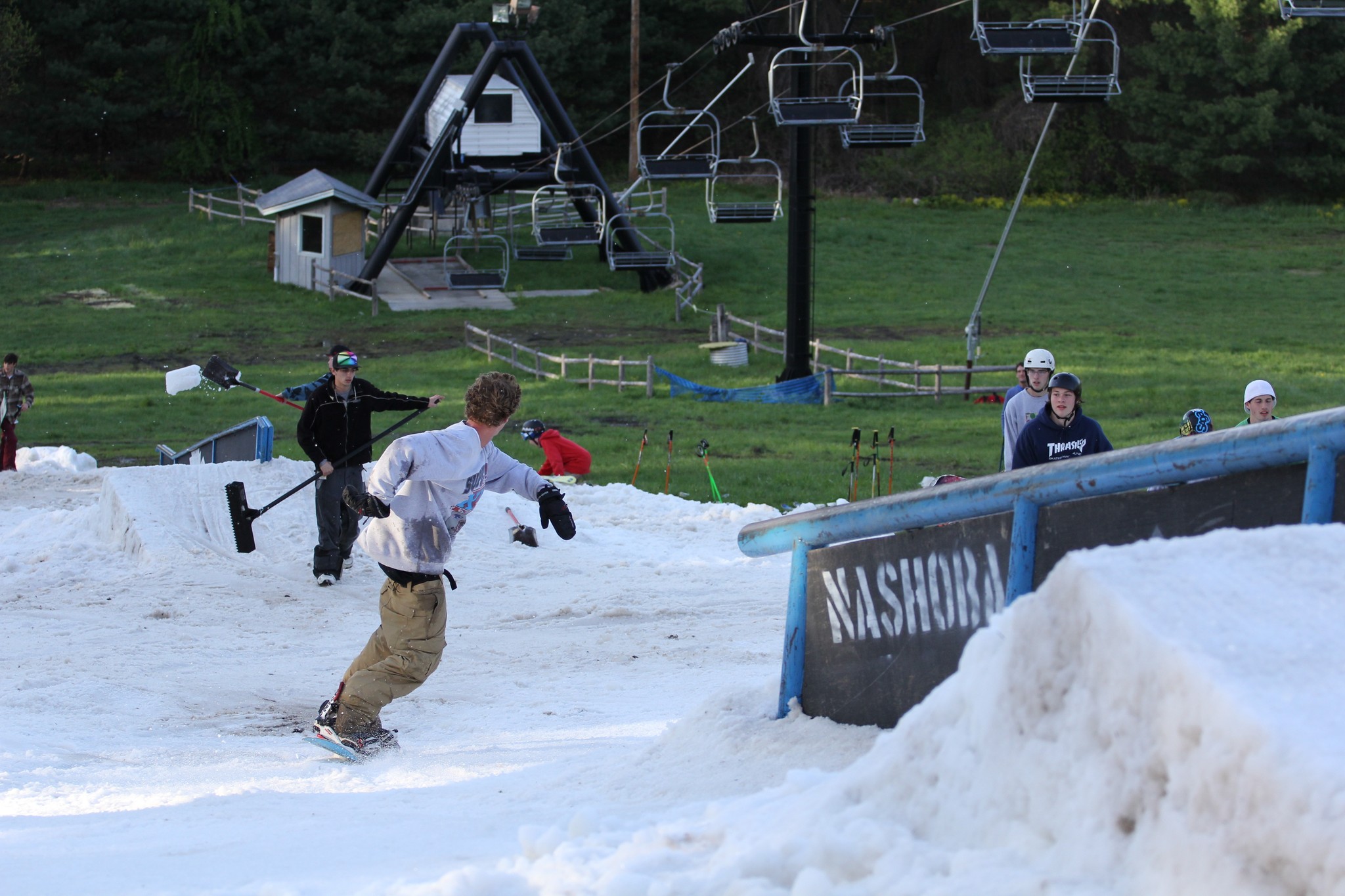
202,355,306,411
504,506,539,546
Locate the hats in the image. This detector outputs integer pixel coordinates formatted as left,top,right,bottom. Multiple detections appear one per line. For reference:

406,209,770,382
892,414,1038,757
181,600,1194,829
332,351,362,369
325,345,351,356
1244,379,1276,414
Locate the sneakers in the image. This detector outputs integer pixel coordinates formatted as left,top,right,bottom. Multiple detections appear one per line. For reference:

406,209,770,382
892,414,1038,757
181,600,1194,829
317,573,336,586
342,553,354,569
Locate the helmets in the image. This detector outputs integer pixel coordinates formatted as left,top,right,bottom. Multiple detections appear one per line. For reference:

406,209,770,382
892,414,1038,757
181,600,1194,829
1179,408,1213,437
1047,372,1082,401
1024,348,1055,374
521,419,546,440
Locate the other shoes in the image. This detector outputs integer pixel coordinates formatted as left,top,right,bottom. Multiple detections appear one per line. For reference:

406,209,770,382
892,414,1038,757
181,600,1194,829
317,699,382,756
313,689,402,750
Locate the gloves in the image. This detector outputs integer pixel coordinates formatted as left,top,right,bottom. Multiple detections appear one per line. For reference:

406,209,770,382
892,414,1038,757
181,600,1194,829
537,487,576,541
342,484,391,519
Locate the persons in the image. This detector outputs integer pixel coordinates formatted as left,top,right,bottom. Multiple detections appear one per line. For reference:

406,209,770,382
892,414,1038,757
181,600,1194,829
1002,362,1030,437
312,370,578,759
1011,371,1114,471
1004,348,1056,474
1173,408,1214,440
276,345,351,400
0,352,34,473
521,420,592,483
1234,380,1283,430
296,351,446,587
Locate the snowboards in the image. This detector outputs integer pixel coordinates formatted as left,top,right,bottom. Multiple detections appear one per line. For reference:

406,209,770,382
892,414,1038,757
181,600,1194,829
303,724,402,763
